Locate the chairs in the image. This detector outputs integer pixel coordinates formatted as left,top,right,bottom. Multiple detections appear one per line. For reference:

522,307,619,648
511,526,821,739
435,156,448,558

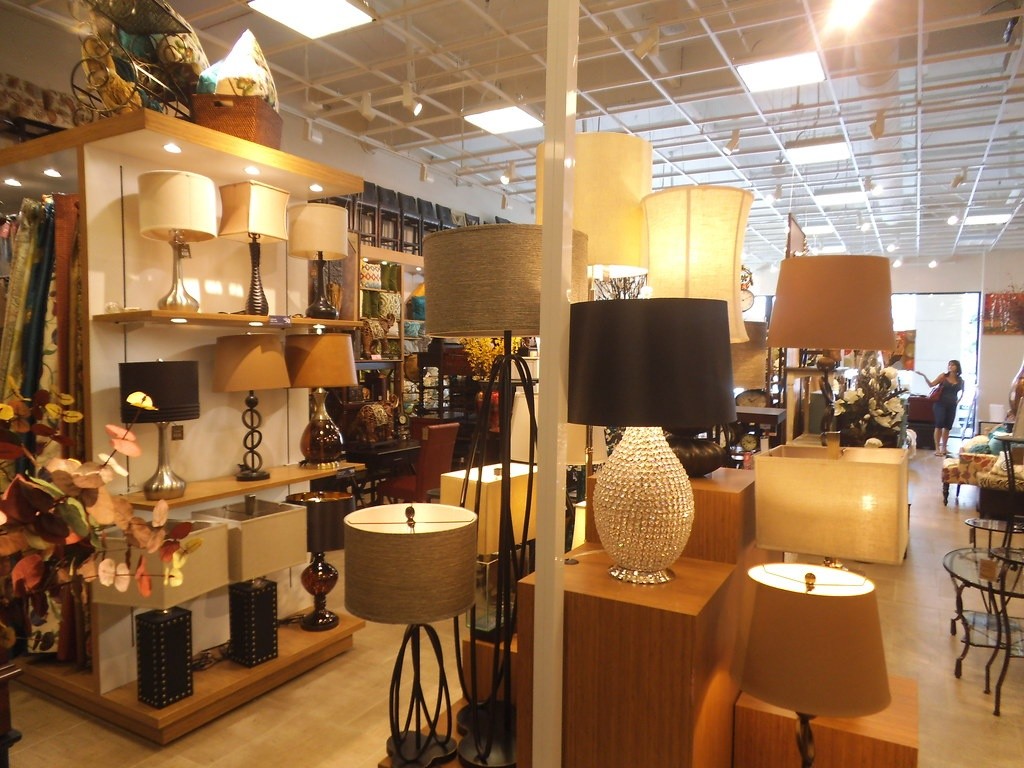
376,423,459,505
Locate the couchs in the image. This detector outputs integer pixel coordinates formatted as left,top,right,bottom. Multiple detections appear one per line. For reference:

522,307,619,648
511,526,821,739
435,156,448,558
942,425,1010,505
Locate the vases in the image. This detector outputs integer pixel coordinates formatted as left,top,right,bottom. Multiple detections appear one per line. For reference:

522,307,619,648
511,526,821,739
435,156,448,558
476,379,502,432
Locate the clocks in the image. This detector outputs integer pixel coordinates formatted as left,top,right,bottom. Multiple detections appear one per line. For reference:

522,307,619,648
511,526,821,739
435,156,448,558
736,389,773,409
740,435,757,451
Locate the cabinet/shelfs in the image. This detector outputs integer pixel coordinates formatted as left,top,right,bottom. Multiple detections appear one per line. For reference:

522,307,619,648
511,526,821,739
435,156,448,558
0,106,366,747
940,433,1024,716
359,257,479,438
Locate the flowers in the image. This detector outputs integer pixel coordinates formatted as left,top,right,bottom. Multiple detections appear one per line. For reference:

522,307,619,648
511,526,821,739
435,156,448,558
1,376,206,649
831,366,909,446
461,334,524,383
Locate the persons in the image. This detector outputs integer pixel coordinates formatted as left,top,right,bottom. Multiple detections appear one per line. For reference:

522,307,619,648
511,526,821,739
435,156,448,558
916,360,964,456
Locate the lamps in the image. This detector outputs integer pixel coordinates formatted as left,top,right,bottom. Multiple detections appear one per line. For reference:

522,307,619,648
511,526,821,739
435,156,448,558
122,33,966,767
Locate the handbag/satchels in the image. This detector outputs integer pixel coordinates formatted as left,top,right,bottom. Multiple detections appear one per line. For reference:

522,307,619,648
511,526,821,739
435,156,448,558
925,375,944,402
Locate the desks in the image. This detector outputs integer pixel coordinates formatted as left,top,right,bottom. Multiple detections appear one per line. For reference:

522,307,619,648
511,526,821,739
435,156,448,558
348,438,421,509
